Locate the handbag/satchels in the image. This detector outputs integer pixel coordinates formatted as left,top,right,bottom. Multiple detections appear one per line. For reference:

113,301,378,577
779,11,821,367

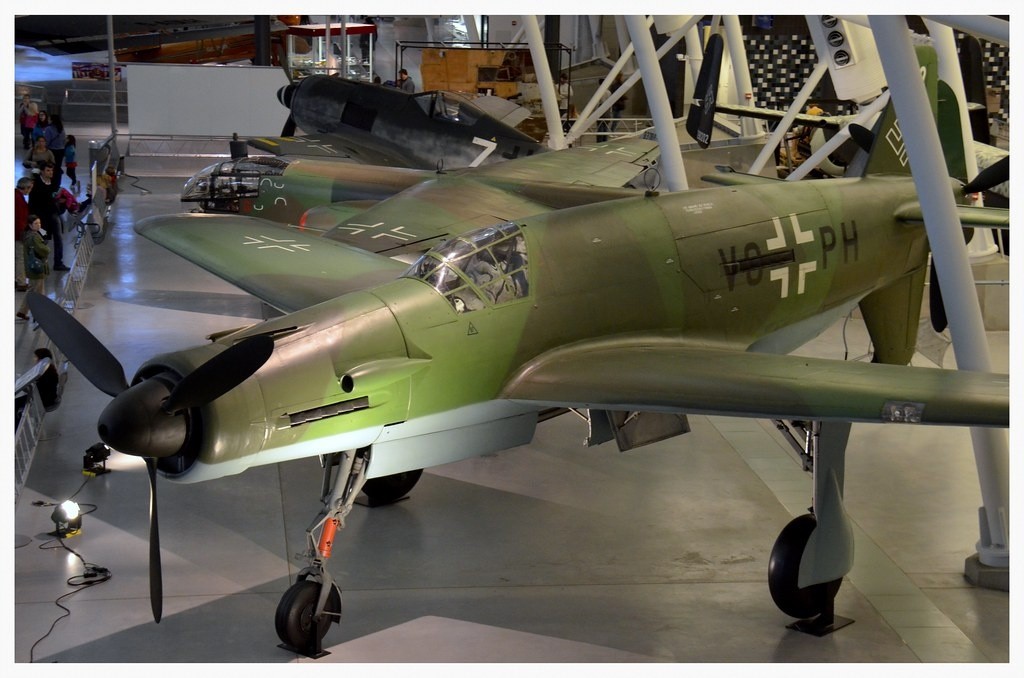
23,148,32,169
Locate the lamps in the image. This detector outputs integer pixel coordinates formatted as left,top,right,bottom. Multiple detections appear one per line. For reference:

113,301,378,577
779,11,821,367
53,501,98,578
82,442,112,478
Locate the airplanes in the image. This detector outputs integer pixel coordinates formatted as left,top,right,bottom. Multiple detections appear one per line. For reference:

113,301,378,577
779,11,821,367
24,44,1010,658
180,38,796,320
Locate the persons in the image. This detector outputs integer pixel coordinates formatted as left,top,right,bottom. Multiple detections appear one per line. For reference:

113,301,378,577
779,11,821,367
560,104,579,148
397,69,415,94
17,214,52,322
790,102,824,153
15,348,60,434
19,94,118,216
358,18,379,65
610,71,628,132
374,77,381,83
556,73,575,121
15,177,34,292
28,162,72,272
597,79,613,144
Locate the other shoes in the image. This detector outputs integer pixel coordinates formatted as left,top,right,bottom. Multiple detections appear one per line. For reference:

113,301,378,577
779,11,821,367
17,286,29,292
54,265,70,271
17,312,29,320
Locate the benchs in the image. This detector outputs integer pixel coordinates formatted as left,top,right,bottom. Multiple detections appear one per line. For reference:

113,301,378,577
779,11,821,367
450,236,528,310
